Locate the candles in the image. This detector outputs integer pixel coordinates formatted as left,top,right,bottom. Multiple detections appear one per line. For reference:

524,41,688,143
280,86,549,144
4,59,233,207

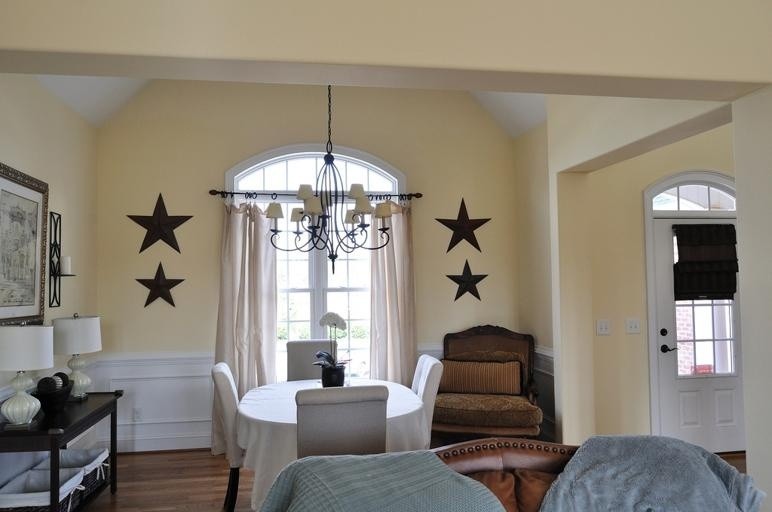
59,253,72,275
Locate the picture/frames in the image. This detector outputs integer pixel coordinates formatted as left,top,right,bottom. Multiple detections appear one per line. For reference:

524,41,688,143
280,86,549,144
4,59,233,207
0,163,50,328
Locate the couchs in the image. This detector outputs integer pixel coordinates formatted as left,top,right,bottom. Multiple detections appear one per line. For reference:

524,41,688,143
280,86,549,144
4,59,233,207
277,435,739,511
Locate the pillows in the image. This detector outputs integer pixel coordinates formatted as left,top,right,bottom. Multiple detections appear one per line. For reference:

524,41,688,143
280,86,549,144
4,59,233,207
438,358,523,396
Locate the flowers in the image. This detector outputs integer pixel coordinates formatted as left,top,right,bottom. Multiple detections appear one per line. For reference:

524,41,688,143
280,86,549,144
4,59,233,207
313,311,352,366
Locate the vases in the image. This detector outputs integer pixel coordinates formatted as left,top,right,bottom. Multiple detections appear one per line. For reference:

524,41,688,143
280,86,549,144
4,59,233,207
321,365,345,387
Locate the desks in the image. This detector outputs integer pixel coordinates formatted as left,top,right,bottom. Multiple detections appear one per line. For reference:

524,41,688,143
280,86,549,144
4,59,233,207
238,376,426,510
0,388,125,511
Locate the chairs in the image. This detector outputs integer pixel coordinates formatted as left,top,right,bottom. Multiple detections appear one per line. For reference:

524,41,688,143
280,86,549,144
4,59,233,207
284,338,338,382
431,322,544,446
294,384,389,459
211,361,246,511
412,351,445,435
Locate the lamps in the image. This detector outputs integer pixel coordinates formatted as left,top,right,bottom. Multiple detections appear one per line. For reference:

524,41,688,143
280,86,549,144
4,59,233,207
0,323,54,426
265,86,395,275
55,315,104,400
